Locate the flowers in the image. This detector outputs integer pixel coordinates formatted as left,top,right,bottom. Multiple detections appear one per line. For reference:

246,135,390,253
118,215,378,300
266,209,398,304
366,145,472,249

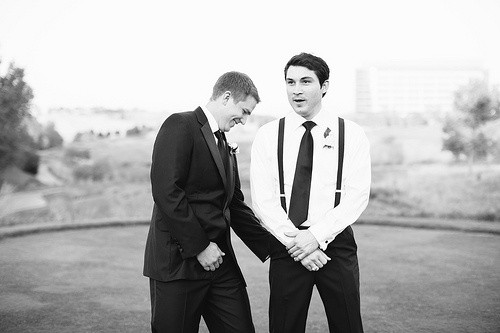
323,126,336,147
229,139,243,157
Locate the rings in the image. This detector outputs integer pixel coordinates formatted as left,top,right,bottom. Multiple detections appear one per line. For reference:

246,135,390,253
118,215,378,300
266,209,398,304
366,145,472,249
312,266,319,271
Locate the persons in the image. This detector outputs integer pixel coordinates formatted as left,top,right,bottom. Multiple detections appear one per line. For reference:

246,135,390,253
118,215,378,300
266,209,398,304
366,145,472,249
143,71,274,333
250,53,373,333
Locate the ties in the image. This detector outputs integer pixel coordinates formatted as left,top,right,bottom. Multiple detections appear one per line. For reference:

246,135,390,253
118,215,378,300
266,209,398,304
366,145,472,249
214,130,226,158
288,121,316,228
